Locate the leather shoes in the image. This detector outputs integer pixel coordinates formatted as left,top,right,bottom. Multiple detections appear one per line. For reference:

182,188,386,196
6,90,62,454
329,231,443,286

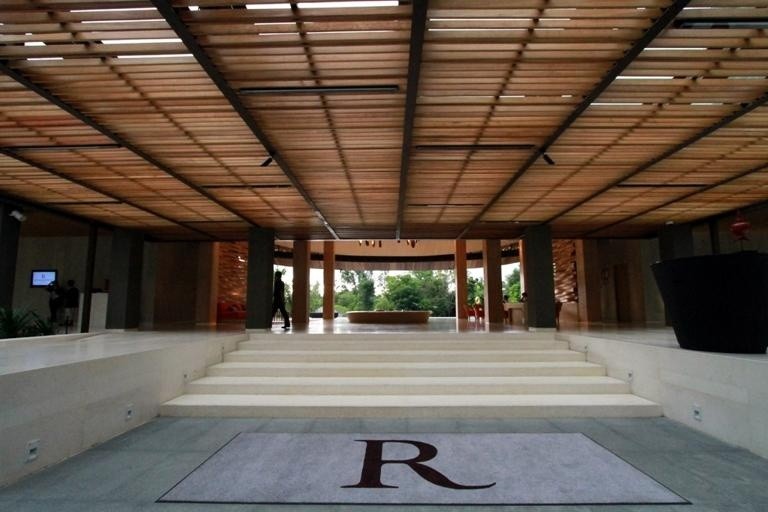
282,325,290,328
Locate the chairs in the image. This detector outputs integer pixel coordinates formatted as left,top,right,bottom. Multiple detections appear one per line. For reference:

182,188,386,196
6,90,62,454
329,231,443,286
555,302,563,325
463,303,508,321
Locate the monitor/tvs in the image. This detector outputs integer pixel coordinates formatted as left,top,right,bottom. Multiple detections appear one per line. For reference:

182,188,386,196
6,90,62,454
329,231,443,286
29,269,57,288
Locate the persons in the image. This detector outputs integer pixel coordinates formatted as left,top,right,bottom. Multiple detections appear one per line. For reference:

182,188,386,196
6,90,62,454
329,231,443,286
272,271,290,328
503,295,508,303
45,279,79,325
521,292,527,302
